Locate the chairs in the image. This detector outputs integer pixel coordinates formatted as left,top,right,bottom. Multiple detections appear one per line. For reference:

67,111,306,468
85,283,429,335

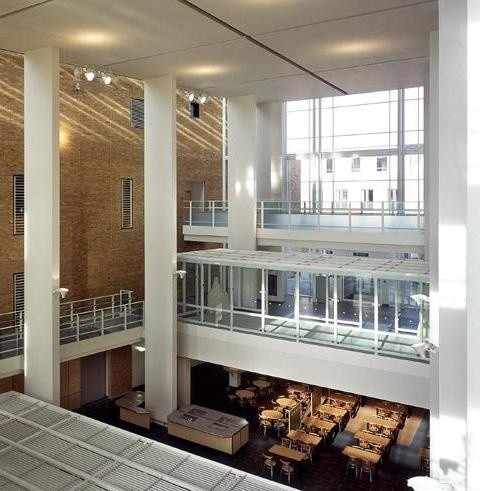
229,376,430,482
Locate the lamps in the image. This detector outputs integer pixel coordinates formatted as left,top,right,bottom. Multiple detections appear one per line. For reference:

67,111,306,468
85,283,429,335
410,292,435,361
83,67,206,105
175,269,187,280
55,287,69,299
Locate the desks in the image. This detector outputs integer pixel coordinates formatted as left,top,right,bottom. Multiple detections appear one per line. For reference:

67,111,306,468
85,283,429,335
167,405,249,453
115,386,151,428
